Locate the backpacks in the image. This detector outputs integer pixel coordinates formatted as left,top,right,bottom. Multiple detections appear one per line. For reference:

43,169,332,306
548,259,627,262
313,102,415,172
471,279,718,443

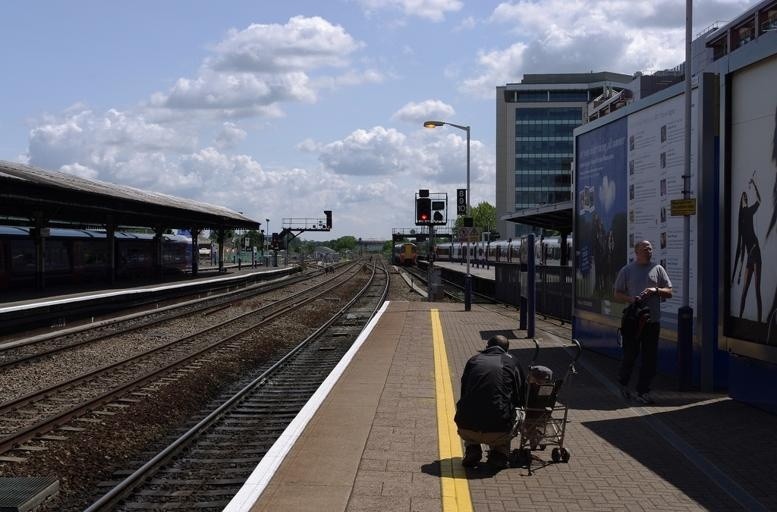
617,297,650,345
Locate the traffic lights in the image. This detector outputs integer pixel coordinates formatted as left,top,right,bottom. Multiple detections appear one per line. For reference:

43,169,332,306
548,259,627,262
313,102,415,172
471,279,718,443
417,198,431,220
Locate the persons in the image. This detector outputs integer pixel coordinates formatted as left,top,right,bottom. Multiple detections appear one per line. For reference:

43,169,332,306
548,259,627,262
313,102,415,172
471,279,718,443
729,177,763,323
452,331,525,470
612,239,676,406
518,364,553,437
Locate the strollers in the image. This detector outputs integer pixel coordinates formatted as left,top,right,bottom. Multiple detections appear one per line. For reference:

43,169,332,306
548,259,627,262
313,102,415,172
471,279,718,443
512,338,584,476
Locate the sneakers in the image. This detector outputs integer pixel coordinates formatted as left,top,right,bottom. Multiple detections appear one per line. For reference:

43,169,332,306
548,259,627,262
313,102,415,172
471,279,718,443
486,451,509,467
615,383,655,405
460,441,484,467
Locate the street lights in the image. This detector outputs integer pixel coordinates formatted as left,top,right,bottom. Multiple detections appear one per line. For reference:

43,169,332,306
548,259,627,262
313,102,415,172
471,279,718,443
424,121,471,311
266,218,270,251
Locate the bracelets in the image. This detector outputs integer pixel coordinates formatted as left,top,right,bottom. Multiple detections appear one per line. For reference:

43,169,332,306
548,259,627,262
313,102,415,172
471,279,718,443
655,286,660,294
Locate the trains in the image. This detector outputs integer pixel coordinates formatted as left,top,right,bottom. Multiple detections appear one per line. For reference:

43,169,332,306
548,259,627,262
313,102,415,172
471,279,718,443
395,243,418,267
0,224,192,268
431,234,575,308
